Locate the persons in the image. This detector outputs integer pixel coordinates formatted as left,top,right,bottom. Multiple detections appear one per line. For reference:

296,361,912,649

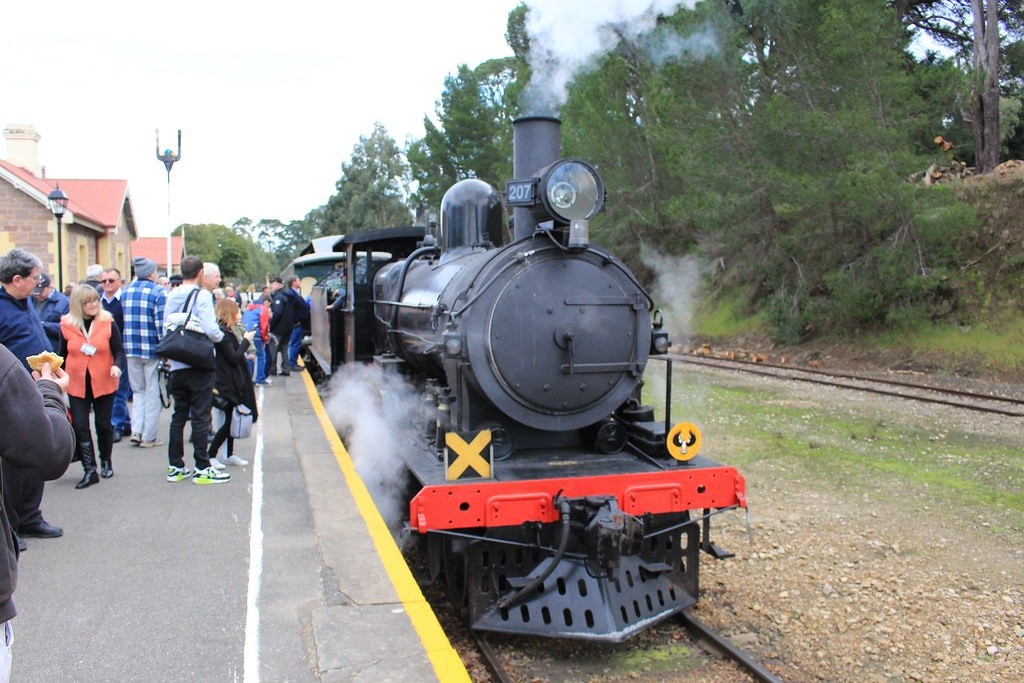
327,268,346,310
0,250,65,550
0,340,75,683
29,274,69,358
162,256,230,485
208,299,256,470
61,256,311,446
56,284,127,488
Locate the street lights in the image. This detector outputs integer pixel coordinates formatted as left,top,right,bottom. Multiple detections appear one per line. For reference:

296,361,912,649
48,182,71,293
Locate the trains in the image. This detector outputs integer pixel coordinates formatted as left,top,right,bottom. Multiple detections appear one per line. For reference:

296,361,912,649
277,117,751,641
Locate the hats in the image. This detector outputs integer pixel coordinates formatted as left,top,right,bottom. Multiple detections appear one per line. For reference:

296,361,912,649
32,271,50,296
133,256,156,278
270,276,281,283
86,264,103,277
170,275,183,284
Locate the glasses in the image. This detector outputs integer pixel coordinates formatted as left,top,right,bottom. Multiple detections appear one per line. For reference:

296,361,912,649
83,299,98,306
171,283,181,287
30,274,40,281
102,279,114,283
163,282,170,285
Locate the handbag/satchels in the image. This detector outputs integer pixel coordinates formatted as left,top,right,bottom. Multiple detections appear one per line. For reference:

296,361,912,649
155,289,215,370
213,396,230,410
230,404,253,439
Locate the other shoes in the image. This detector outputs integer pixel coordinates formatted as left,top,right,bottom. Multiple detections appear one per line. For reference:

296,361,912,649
15,533,27,551
290,365,304,368
16,521,63,537
189,430,217,443
255,379,273,387
112,428,132,442
278,371,290,376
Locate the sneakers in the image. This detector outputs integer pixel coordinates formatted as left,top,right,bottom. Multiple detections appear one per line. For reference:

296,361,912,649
209,458,226,469
167,466,190,481
192,466,230,484
129,432,164,447
222,455,248,466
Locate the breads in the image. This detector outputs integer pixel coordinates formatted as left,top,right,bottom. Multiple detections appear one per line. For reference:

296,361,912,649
26,350,64,372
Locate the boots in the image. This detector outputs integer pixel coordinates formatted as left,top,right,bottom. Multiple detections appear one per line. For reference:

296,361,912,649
76,430,99,489
97,426,114,478
